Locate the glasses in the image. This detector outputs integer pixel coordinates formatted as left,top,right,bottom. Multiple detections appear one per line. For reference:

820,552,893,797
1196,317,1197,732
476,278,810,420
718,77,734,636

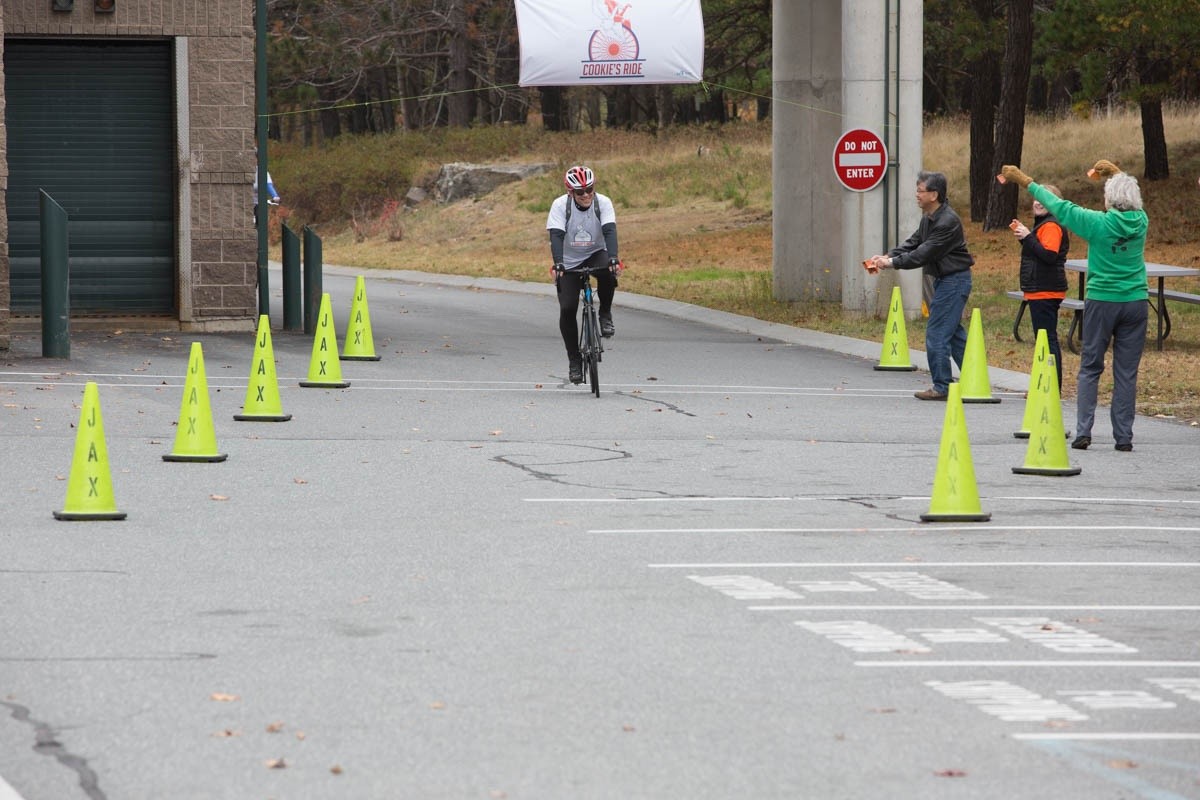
572,185,593,195
917,188,933,194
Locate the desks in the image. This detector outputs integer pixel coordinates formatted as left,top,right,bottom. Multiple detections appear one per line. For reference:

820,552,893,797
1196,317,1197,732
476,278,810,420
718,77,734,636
1062,258,1200,349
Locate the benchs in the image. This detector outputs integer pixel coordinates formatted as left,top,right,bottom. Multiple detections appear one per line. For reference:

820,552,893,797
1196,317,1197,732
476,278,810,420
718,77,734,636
1148,289,1200,341
1008,290,1087,355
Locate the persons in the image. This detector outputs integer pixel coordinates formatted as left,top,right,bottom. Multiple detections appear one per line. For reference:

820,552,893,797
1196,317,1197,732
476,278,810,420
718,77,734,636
1001,160,1149,451
253,145,280,288
870,172,975,401
545,167,620,383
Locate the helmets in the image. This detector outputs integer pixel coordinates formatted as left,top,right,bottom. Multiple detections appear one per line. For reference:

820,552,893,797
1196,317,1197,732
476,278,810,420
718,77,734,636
564,165,594,191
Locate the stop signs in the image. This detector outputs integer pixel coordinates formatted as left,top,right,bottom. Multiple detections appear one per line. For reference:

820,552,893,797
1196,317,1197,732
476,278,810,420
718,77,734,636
832,130,886,194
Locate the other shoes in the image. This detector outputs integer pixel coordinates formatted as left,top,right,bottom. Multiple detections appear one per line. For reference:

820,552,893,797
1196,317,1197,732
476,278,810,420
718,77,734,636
1115,443,1132,451
1071,436,1091,449
913,388,948,401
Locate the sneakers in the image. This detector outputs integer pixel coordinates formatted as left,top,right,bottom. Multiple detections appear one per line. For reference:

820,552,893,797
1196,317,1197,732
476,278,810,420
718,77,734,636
568,359,583,382
599,315,614,337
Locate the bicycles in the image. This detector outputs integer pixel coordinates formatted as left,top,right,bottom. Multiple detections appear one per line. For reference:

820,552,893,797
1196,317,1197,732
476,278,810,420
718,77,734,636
554,264,621,399
253,200,281,290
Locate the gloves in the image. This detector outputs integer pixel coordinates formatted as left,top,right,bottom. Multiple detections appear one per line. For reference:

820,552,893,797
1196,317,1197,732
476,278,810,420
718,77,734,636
1002,163,1033,188
1094,159,1124,177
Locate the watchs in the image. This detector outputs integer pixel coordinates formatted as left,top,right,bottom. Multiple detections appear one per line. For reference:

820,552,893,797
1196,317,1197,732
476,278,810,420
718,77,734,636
888,258,893,267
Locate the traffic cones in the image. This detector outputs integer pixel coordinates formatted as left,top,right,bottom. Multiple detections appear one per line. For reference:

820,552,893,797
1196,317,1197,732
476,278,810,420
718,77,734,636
876,284,919,372
1011,328,1082,476
957,308,1003,403
236,312,293,422
53,379,127,520
339,276,384,362
920,383,992,522
297,291,350,388
163,341,227,462
